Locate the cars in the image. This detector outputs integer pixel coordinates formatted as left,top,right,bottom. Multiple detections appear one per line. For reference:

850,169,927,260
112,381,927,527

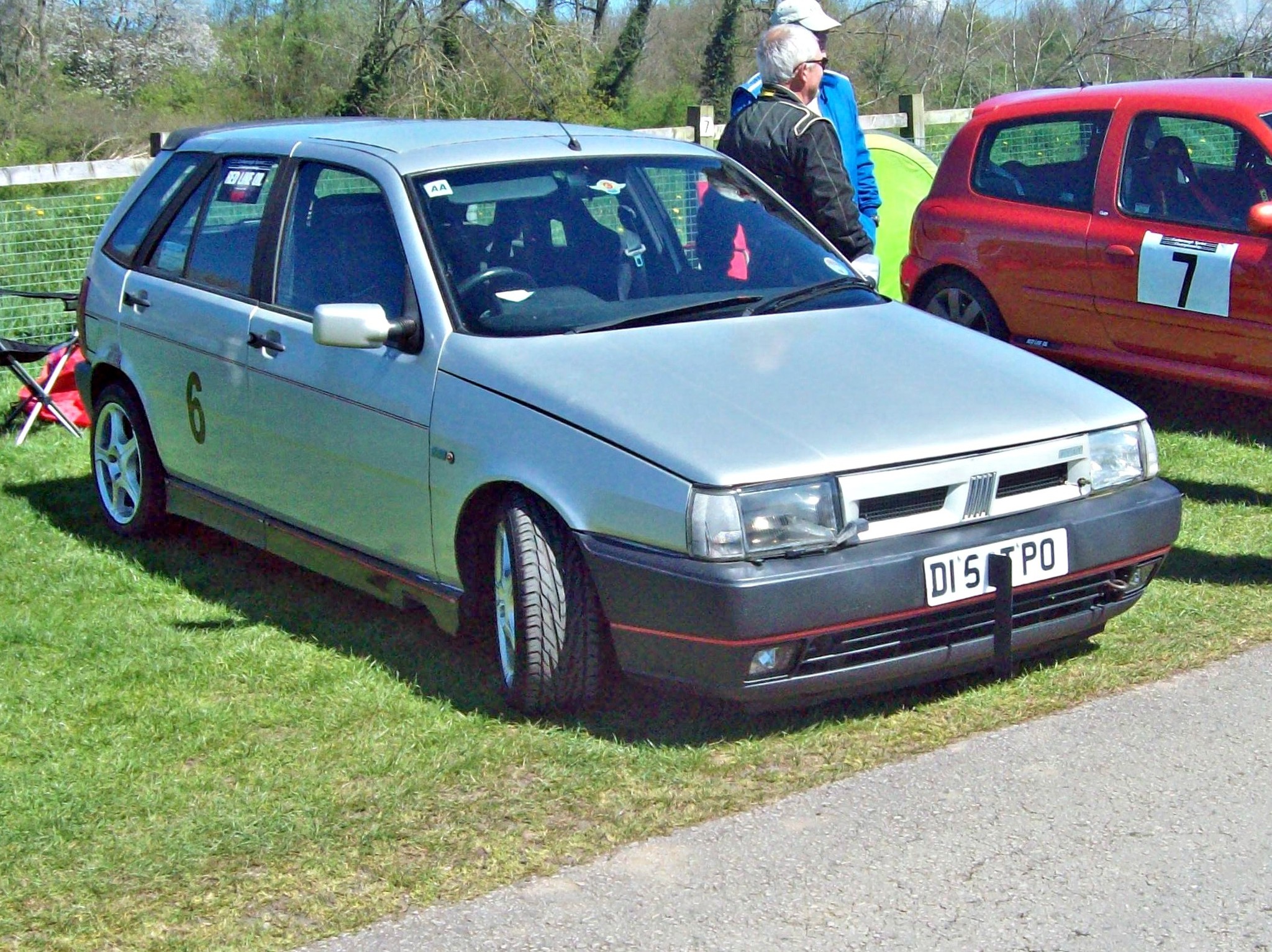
900,77,1271,396
74,116,1184,721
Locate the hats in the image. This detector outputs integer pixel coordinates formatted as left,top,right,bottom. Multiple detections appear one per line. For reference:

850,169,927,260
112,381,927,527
769,0,842,32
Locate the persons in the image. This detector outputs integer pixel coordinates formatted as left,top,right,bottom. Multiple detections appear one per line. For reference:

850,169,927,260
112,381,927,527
729,0,882,245
695,23,874,290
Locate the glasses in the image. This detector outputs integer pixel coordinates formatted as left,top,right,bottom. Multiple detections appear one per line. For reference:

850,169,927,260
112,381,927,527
793,57,829,74
